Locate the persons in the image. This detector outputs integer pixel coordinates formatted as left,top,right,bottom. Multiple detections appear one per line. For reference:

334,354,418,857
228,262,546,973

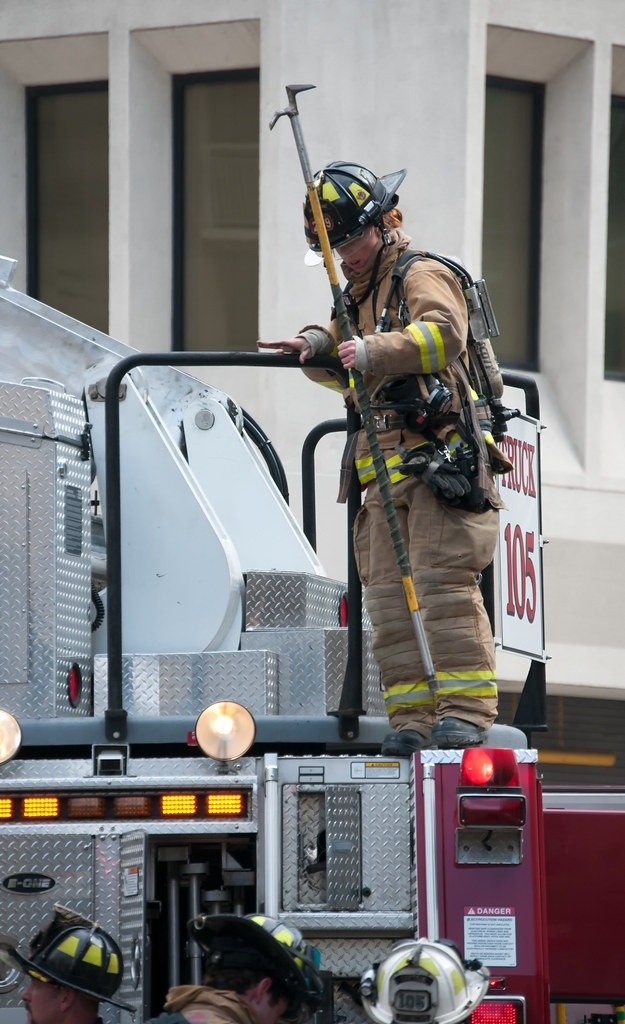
8,905,138,1024
359,937,490,1024
149,913,324,1024
255,161,520,755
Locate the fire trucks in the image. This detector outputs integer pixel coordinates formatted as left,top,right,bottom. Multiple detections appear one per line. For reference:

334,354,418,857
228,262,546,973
1,256,625,1024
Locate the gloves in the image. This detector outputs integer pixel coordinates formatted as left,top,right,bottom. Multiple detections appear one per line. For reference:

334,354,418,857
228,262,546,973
391,441,472,500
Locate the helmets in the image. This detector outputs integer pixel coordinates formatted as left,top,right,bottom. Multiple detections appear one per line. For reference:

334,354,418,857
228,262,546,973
185,912,325,1022
0,925,138,1015
357,935,491,1024
303,155,407,271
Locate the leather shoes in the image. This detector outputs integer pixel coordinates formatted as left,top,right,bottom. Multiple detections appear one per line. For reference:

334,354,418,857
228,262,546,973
380,729,437,757
431,714,487,745
